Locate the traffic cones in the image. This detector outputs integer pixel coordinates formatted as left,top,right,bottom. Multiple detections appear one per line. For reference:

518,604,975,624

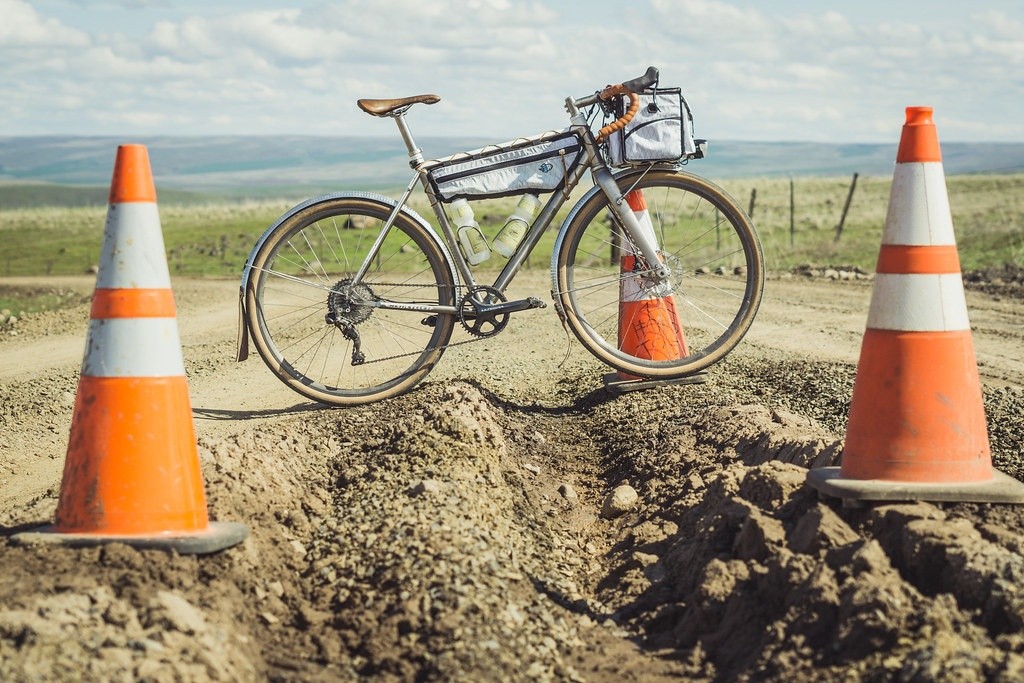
6,142,250,560
599,190,713,396
806,104,1024,505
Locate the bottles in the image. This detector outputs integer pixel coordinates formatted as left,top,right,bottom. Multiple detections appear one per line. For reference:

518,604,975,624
491,193,548,259
449,195,492,265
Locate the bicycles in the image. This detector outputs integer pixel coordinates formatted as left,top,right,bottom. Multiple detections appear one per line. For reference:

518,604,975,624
230,64,768,410
